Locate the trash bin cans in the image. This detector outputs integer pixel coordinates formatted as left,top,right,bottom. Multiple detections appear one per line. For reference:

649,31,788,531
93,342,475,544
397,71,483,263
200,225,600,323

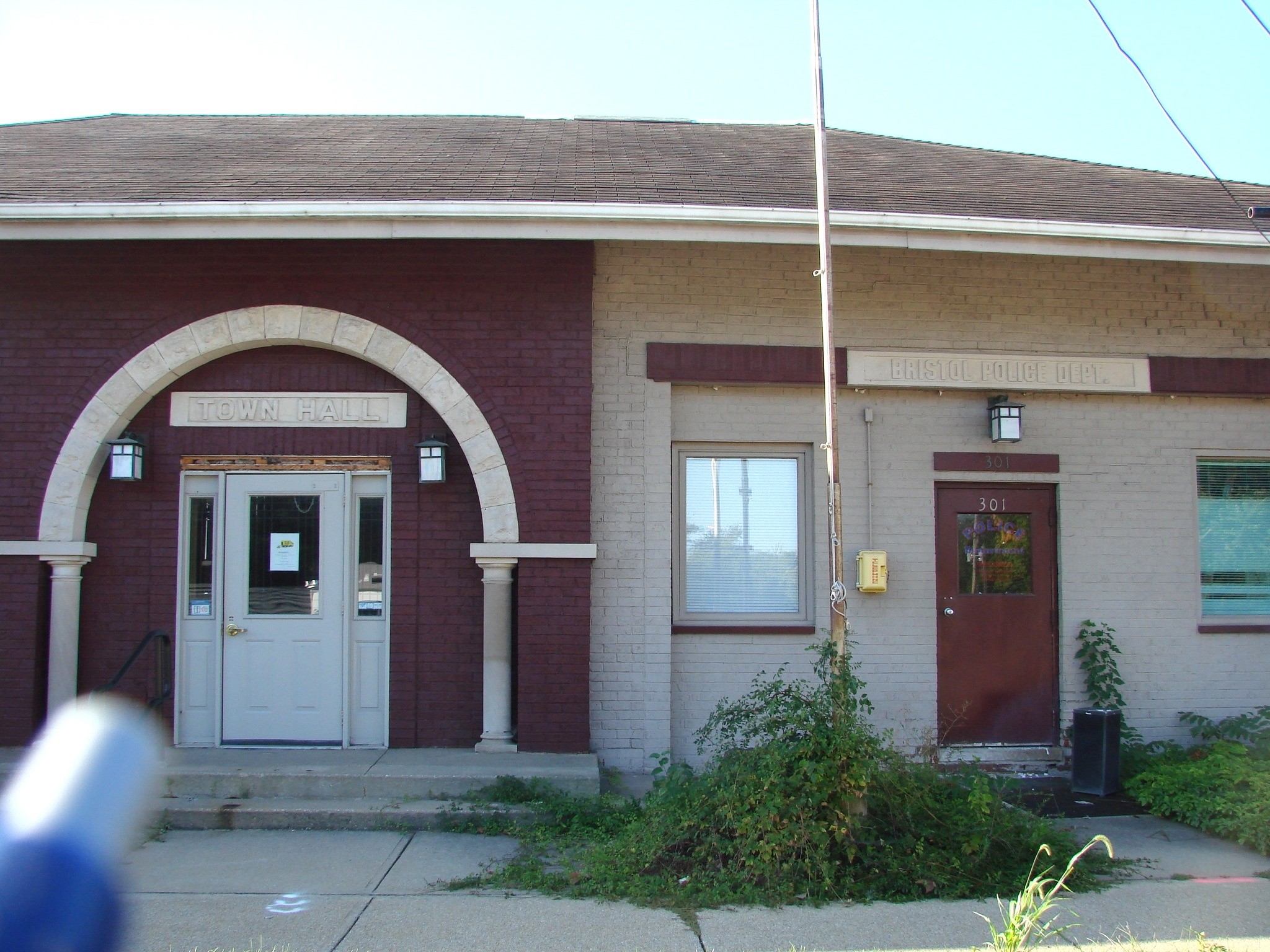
1071,707,1122,798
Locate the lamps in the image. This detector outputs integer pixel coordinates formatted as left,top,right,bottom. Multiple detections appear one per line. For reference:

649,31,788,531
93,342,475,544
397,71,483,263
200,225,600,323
105,431,145,482
414,433,449,483
985,394,1026,443
